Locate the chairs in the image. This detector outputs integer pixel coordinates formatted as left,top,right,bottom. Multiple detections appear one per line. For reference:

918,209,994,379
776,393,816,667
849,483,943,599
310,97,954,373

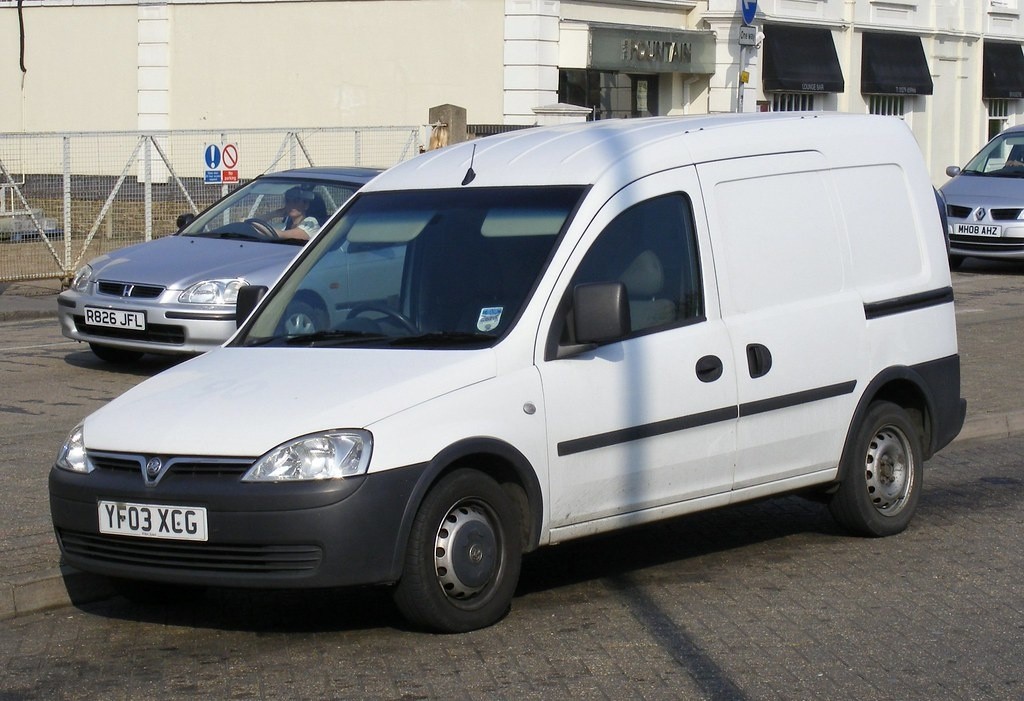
618,249,676,332
282,192,326,230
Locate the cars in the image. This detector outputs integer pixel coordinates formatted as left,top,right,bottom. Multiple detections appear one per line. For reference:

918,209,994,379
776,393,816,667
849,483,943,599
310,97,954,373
936,124,1024,269
57,167,391,364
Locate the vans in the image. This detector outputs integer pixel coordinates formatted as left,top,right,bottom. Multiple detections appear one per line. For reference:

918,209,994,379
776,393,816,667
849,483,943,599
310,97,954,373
49,110,968,633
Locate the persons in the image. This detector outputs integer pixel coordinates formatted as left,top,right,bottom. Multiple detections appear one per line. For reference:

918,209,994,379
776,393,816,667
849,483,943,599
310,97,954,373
252,187,320,240
1005,160,1024,167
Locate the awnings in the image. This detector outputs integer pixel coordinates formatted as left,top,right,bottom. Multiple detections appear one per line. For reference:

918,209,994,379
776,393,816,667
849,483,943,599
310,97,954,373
861,32,933,95
763,24,844,93
982,42,1024,99
